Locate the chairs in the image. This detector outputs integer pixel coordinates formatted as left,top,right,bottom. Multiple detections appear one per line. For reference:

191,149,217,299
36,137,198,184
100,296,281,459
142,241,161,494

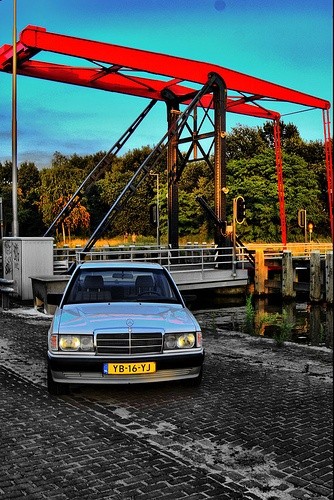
126,275,162,301
75,274,111,304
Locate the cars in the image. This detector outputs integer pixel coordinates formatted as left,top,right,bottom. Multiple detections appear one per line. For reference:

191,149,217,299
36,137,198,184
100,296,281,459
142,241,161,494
47,259,205,396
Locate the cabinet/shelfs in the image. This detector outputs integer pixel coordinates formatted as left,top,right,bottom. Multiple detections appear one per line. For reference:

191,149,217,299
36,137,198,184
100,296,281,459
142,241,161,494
2,237,55,305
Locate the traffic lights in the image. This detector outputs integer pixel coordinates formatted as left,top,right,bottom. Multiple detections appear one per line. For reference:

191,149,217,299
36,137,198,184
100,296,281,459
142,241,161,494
308,223,313,233
236,197,246,225
